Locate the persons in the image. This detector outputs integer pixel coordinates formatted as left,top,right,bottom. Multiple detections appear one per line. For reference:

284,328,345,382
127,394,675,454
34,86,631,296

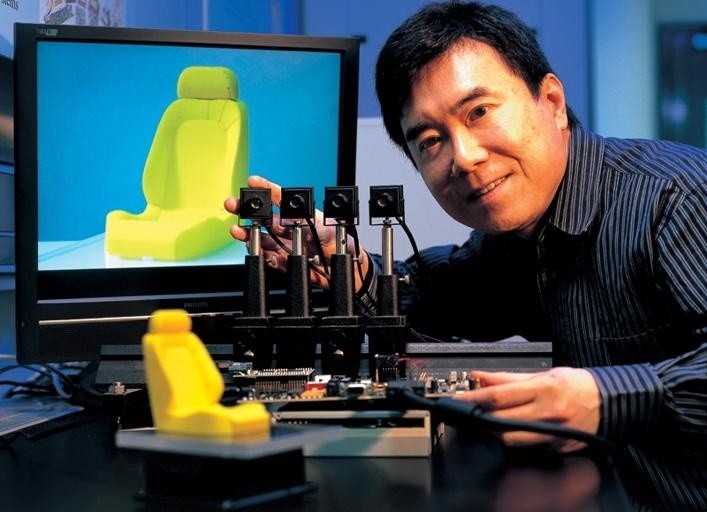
225,1,707,512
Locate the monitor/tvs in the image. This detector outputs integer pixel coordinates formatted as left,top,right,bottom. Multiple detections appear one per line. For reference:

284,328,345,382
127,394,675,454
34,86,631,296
12,20,366,367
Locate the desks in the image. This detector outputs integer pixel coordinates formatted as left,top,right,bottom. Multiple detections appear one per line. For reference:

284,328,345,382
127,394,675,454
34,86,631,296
0,369,631,512
